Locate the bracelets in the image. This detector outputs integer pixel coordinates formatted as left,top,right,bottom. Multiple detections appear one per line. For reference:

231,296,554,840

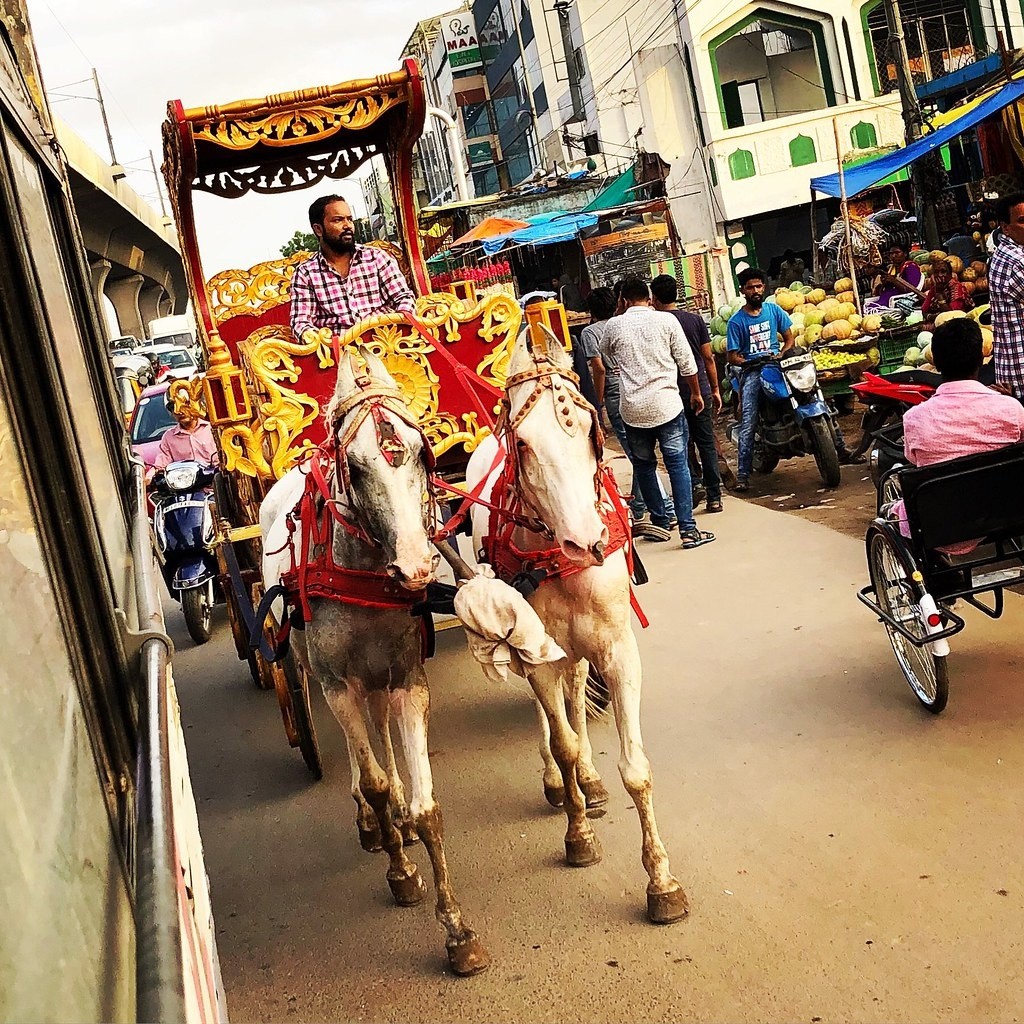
938,300,946,304
713,385,718,388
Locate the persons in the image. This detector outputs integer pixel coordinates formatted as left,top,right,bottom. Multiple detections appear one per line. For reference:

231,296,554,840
988,193,1024,404
818,246,837,281
922,260,976,322
725,269,867,491
551,273,591,311
650,274,723,512
145,386,220,492
777,250,805,287
599,279,717,548
289,194,416,345
525,295,607,431
146,353,171,379
580,287,677,526
867,242,921,314
887,318,1024,558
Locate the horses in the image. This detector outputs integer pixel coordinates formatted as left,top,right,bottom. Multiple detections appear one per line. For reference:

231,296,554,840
257,321,690,977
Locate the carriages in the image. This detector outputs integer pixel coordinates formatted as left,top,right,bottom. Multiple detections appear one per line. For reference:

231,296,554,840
156,56,690,978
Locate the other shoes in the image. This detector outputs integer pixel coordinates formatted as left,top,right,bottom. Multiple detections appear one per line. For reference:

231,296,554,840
719,461,738,492
838,448,866,464
627,501,645,521
734,481,748,492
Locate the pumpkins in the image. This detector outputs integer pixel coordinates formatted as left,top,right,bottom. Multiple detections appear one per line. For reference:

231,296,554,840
904,304,994,374
763,277,905,348
909,249,989,296
709,296,746,353
867,347,880,367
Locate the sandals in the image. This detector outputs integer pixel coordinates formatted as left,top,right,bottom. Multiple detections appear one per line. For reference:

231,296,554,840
706,499,723,512
680,529,715,548
692,483,705,510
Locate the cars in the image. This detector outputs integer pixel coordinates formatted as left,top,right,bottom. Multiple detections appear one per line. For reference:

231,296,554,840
99,317,206,531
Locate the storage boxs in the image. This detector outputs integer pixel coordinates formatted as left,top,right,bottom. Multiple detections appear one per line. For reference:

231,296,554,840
819,374,855,398
879,331,918,364
878,361,905,375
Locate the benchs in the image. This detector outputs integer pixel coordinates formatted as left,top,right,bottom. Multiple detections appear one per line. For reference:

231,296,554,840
205,237,418,367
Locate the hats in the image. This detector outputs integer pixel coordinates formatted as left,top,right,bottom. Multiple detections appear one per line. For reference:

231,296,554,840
163,388,189,416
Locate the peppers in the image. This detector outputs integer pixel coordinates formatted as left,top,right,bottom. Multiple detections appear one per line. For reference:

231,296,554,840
811,350,868,370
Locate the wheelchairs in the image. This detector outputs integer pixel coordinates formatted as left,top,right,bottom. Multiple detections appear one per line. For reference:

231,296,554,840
856,418,1024,717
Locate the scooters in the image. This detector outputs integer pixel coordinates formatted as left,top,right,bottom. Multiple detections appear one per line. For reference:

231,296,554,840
129,449,227,645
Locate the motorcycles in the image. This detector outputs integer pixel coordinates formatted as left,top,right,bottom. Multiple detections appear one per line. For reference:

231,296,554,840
850,306,995,490
725,334,841,488
